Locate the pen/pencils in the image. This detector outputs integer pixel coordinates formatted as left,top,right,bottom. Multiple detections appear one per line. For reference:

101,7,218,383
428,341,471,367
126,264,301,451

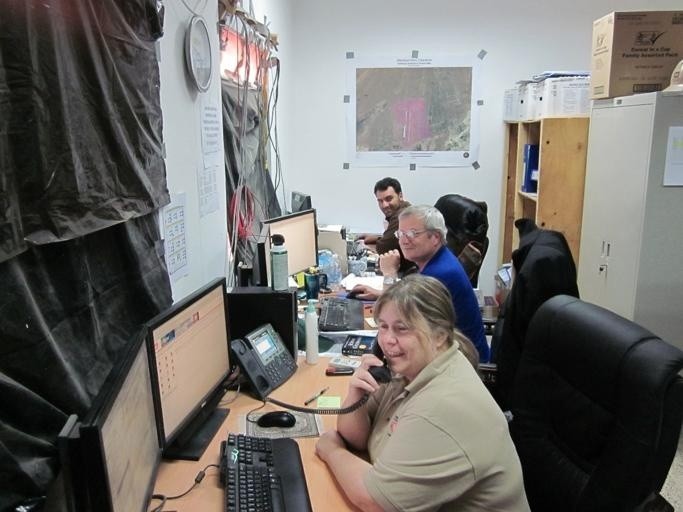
304,387,330,405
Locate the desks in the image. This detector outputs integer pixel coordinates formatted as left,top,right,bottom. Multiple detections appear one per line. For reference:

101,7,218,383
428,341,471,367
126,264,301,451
298,280,379,329
150,348,362,512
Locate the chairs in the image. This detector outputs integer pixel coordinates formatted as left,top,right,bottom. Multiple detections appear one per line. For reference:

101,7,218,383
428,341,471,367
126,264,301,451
477,229,579,373
504,295,683,512
434,195,489,289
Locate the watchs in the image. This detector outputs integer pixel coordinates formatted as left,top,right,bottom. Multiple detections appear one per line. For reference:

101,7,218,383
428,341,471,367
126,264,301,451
382,275,396,286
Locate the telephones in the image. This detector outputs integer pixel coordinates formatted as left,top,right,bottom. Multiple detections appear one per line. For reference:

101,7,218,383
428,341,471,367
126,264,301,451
368,332,391,384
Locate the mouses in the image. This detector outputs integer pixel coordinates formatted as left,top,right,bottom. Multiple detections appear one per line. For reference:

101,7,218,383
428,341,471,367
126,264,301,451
346,291,364,299
258,411,296,427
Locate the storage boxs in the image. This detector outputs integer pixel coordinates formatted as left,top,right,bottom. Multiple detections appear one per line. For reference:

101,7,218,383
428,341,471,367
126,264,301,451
504,88,517,120
591,10,683,100
534,75,592,115
517,82,537,120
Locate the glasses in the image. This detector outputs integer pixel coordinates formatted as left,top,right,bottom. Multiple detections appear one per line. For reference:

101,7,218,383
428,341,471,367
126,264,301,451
394,230,426,240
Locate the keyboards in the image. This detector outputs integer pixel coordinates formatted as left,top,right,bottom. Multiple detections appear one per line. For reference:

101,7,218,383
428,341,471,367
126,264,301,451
226,434,312,512
318,297,351,331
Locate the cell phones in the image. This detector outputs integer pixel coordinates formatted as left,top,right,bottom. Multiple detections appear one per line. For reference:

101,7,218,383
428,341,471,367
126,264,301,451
326,367,354,375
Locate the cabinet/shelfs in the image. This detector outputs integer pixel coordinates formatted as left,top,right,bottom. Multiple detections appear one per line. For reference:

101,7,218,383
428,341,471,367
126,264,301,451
499,116,589,268
578,91,683,350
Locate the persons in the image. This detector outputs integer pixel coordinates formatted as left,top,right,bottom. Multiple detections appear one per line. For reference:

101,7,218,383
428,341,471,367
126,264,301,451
363,176,413,272
314,270,533,512
348,203,490,364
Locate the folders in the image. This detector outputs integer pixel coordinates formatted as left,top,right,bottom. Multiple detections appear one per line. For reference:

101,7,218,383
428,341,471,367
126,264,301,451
522,144,538,193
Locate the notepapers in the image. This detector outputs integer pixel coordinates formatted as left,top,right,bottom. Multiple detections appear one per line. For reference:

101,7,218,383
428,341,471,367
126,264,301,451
317,396,340,409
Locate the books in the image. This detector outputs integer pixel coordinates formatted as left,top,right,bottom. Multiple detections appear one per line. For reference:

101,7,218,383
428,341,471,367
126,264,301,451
341,334,376,357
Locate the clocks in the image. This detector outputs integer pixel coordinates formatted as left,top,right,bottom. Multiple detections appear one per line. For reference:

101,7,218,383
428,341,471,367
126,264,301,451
185,15,212,92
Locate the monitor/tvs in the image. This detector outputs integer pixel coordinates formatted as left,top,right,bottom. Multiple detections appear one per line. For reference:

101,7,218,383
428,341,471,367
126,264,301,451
237,224,272,287
142,276,232,461
57,326,164,512
292,192,311,213
260,208,318,300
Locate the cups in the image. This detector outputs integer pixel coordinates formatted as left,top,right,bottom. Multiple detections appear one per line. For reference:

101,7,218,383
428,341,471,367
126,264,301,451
304,274,327,299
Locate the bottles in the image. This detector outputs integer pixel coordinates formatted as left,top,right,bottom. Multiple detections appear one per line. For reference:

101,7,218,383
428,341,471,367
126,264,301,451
356,240,367,270
330,254,342,293
269,234,288,291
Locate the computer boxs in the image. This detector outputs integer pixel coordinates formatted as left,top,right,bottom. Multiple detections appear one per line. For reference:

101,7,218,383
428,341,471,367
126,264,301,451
226,286,298,365
317,224,349,276
